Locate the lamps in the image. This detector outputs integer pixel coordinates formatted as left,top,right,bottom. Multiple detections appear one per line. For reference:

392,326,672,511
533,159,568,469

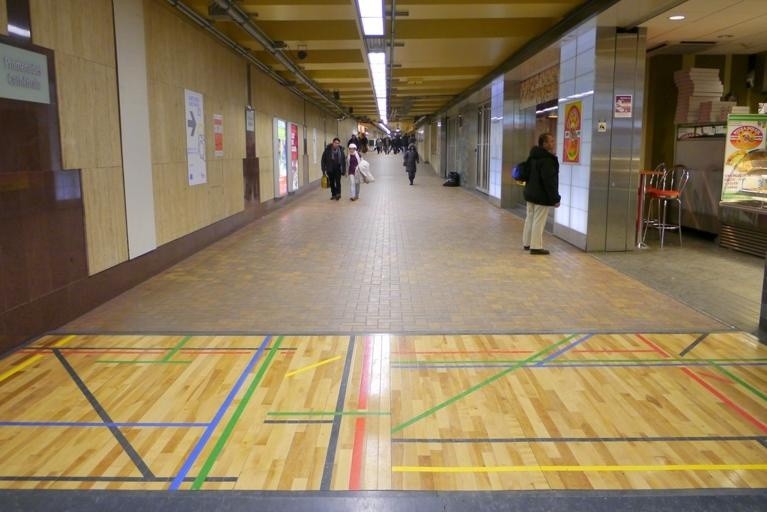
548,109,559,119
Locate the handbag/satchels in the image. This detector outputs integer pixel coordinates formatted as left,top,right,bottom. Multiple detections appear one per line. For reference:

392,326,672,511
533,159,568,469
321,175,330,188
511,162,527,180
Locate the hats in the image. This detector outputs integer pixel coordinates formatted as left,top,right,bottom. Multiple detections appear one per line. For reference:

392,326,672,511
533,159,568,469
349,143,357,149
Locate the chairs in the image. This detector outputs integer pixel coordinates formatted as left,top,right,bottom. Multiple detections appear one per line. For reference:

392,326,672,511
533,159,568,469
642,162,668,242
643,165,690,250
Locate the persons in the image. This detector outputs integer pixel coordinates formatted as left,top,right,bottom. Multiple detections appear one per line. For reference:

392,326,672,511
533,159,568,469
522,132,562,255
320,139,345,200
375,131,417,153
402,144,420,186
347,131,369,154
345,145,365,201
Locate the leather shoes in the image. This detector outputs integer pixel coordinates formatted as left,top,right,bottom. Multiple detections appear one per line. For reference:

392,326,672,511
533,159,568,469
331,195,341,200
531,249,549,255
